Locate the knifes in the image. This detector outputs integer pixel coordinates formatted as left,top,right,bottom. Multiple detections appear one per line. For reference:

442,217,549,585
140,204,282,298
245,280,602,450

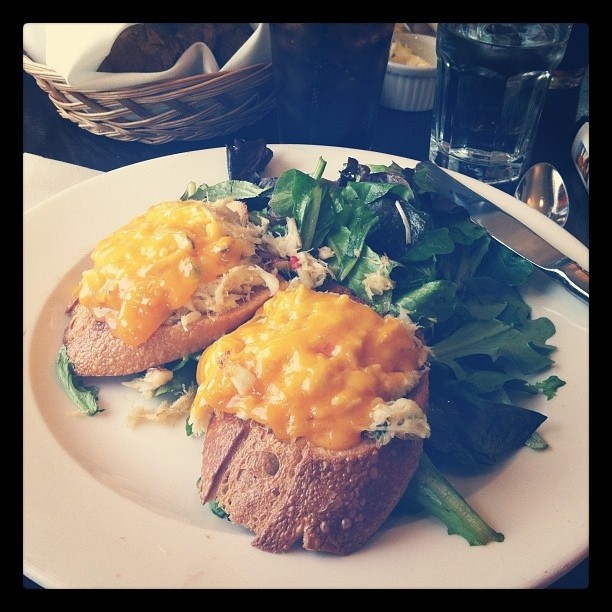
415,161,589,301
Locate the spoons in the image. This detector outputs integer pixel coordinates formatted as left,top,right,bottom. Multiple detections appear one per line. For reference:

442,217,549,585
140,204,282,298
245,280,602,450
513,162,569,227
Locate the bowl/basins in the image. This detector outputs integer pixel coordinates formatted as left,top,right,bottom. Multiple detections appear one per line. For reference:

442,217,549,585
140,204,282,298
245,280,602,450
380,33,438,112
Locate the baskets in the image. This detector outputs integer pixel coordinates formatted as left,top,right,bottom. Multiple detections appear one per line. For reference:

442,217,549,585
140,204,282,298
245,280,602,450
23,23,277,144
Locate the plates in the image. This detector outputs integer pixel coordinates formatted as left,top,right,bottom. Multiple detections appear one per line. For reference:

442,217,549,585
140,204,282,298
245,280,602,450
22,143,588,590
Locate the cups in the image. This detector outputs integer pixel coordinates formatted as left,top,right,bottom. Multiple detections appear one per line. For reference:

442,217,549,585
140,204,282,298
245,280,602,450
428,21,573,185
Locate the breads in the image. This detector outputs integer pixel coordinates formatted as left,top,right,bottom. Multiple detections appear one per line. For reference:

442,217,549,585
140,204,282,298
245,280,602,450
62,199,286,378
190,279,430,556
